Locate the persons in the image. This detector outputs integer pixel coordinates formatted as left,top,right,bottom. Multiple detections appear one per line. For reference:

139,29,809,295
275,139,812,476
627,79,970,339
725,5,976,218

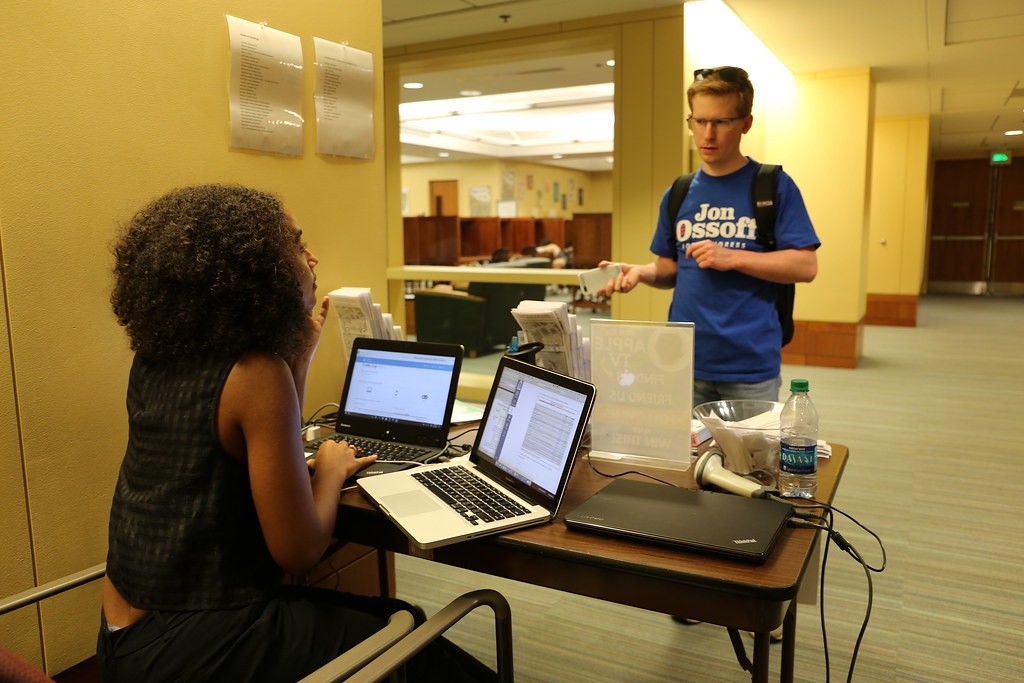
596,64,820,646
92,183,497,683
493,240,576,297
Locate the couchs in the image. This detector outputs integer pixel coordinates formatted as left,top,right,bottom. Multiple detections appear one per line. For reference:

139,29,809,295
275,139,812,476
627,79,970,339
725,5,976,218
468,260,523,344
413,289,506,358
517,255,551,302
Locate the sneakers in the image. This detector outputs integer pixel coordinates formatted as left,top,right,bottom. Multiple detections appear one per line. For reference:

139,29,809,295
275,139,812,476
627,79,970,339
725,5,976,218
749,625,782,643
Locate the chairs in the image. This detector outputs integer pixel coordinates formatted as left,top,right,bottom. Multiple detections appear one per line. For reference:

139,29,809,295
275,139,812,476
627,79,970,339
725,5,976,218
0,562,515,683
572,284,610,315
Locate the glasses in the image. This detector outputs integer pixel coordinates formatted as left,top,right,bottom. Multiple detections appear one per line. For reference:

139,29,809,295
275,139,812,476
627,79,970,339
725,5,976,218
687,116,745,128
694,68,753,96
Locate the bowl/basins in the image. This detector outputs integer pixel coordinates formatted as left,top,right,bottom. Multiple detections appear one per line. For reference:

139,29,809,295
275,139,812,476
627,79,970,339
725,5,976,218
692,399,786,478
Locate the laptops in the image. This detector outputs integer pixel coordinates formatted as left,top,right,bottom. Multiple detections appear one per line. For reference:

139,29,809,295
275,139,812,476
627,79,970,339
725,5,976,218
356,356,598,552
302,337,464,476
563,475,794,563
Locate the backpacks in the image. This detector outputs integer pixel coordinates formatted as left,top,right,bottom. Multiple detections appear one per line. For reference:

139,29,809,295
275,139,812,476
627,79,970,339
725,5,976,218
668,164,796,347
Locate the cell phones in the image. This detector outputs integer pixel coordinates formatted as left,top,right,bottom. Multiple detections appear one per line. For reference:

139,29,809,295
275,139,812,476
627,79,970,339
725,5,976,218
579,264,623,293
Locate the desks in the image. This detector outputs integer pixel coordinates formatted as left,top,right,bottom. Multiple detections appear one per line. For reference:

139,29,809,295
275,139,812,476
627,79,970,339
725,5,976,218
300,408,850,683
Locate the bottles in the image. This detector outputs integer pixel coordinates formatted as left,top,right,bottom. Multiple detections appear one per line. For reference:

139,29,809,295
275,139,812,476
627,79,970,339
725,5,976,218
780,379,818,500
